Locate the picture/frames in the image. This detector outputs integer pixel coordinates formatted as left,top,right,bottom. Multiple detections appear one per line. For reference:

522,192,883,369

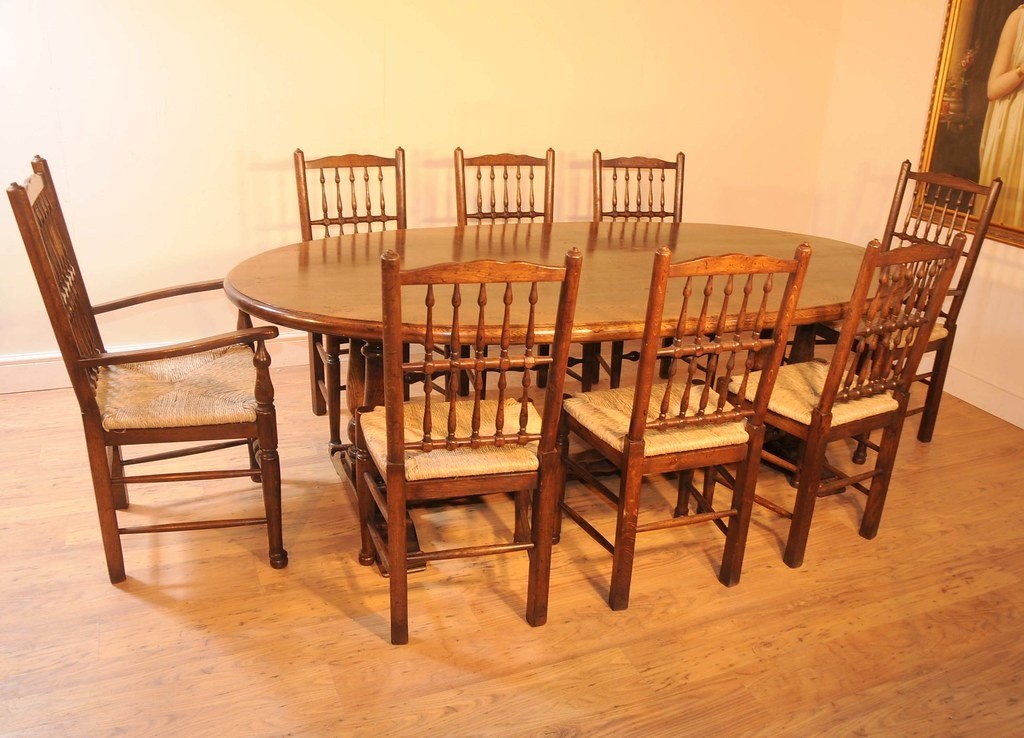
907,0,1024,251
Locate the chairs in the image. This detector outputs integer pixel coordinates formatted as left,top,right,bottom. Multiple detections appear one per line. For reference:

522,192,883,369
6,154,289,586
293,146,409,416
695,232,967,568
355,246,583,645
550,241,812,611
783,159,1003,466
591,149,685,390
453,147,554,395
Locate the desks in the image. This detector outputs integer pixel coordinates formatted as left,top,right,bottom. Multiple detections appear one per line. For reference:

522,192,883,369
223,223,915,567
